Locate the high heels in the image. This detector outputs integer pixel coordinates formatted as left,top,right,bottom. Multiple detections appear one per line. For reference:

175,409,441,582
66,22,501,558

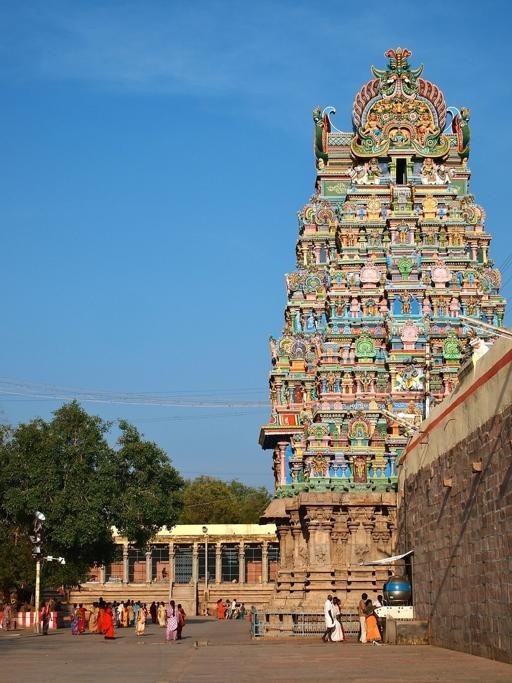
373,640,379,645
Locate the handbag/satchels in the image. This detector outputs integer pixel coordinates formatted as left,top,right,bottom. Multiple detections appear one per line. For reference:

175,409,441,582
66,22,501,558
180,620,185,627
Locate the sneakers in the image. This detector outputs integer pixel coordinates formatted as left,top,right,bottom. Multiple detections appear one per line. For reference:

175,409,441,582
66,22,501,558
322,637,347,642
160,625,167,628
136,633,144,636
43,632,47,635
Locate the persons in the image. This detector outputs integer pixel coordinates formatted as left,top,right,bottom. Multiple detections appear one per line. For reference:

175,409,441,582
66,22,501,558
0,577,257,643
321,592,385,644
466,327,490,360
161,567,168,579
455,334,473,364
